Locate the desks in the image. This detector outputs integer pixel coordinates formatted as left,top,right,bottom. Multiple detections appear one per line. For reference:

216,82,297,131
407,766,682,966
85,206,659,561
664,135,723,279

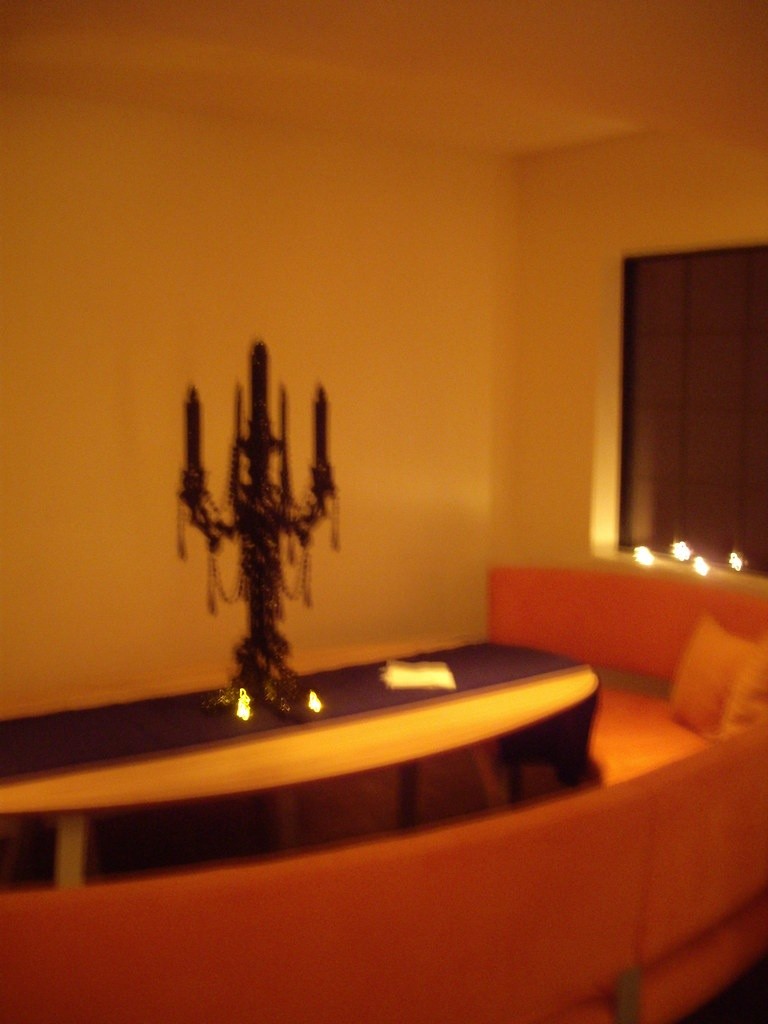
0,635,600,888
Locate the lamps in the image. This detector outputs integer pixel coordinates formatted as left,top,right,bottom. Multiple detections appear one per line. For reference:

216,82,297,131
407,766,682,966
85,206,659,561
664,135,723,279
177,338,338,728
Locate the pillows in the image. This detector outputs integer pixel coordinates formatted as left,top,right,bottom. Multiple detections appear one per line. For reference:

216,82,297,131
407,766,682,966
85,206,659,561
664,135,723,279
670,610,757,739
721,629,768,738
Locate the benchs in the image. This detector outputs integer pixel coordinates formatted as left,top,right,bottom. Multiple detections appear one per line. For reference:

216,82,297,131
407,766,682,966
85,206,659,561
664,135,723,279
0,556,766,1023
